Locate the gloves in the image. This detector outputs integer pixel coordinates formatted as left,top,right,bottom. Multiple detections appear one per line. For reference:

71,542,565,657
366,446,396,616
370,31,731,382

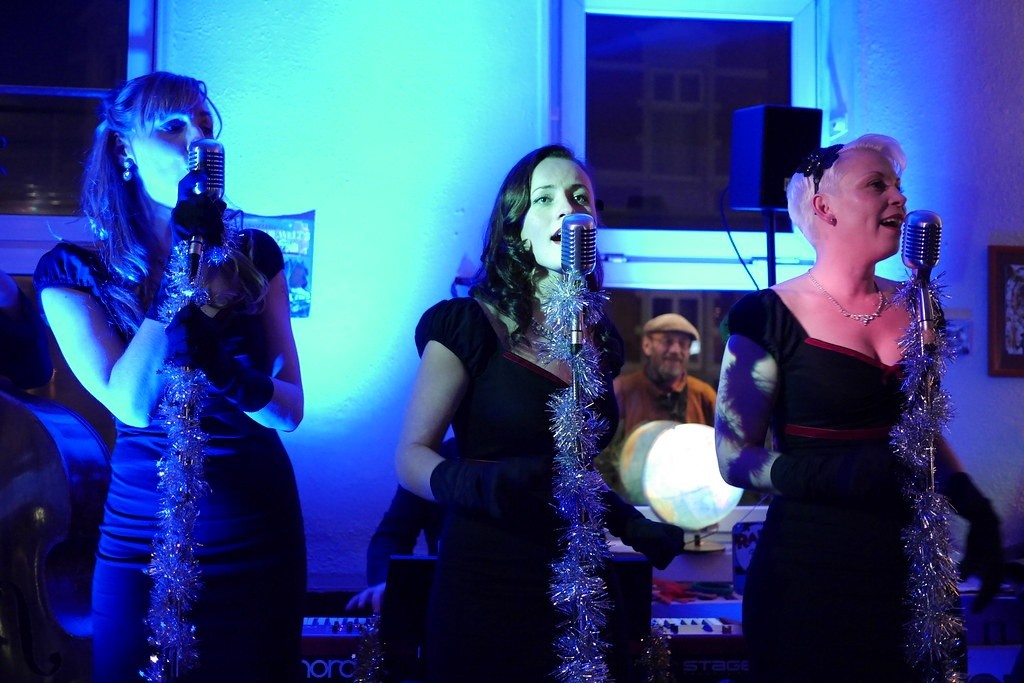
430,458,552,527
769,452,892,512
941,474,1002,610
164,302,275,415
602,491,684,567
171,166,229,247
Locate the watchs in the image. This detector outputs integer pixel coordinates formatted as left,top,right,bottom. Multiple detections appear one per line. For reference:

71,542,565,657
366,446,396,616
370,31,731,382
603,313,721,441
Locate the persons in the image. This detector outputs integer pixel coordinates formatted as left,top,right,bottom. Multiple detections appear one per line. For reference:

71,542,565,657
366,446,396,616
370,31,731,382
394,140,684,683
342,432,450,618
710,134,1010,683
31,67,310,683
0,268,53,390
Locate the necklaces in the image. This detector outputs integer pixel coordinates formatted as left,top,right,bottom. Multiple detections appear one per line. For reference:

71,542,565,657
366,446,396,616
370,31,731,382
530,316,572,344
808,267,889,327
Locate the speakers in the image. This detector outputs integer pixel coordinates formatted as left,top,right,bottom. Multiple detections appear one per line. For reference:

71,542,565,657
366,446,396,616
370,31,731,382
729,104,824,211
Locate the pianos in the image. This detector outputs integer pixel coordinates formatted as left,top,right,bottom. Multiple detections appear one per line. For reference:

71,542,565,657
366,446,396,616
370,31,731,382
296,616,748,683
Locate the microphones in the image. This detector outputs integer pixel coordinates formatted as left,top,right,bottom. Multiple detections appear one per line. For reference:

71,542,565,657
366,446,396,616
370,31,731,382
561,214,598,356
186,139,225,274
901,209,942,350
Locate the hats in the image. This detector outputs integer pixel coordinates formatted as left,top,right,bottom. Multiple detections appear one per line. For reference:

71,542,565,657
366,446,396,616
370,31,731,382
643,313,700,339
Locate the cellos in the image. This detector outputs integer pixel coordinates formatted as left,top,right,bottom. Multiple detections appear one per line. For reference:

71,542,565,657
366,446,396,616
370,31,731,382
0,388,114,683
